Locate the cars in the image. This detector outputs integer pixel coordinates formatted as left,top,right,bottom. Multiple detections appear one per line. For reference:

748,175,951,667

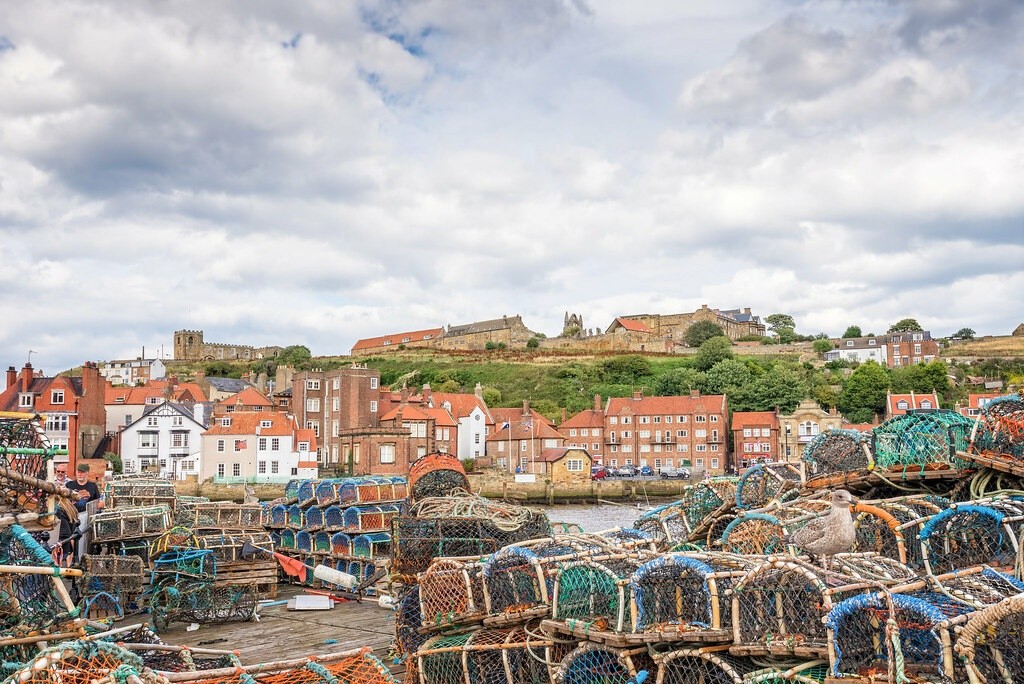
593,463,691,483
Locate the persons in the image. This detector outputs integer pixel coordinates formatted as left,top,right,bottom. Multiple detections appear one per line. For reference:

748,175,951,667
701,464,740,482
52,463,73,489
64,464,100,569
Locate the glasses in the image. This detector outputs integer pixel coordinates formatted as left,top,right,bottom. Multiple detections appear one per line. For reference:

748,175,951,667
56,470,66,474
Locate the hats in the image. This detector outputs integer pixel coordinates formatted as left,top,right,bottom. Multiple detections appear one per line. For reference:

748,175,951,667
77,463,90,472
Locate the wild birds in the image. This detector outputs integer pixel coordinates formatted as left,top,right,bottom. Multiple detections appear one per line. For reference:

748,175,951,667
789,488,859,585
